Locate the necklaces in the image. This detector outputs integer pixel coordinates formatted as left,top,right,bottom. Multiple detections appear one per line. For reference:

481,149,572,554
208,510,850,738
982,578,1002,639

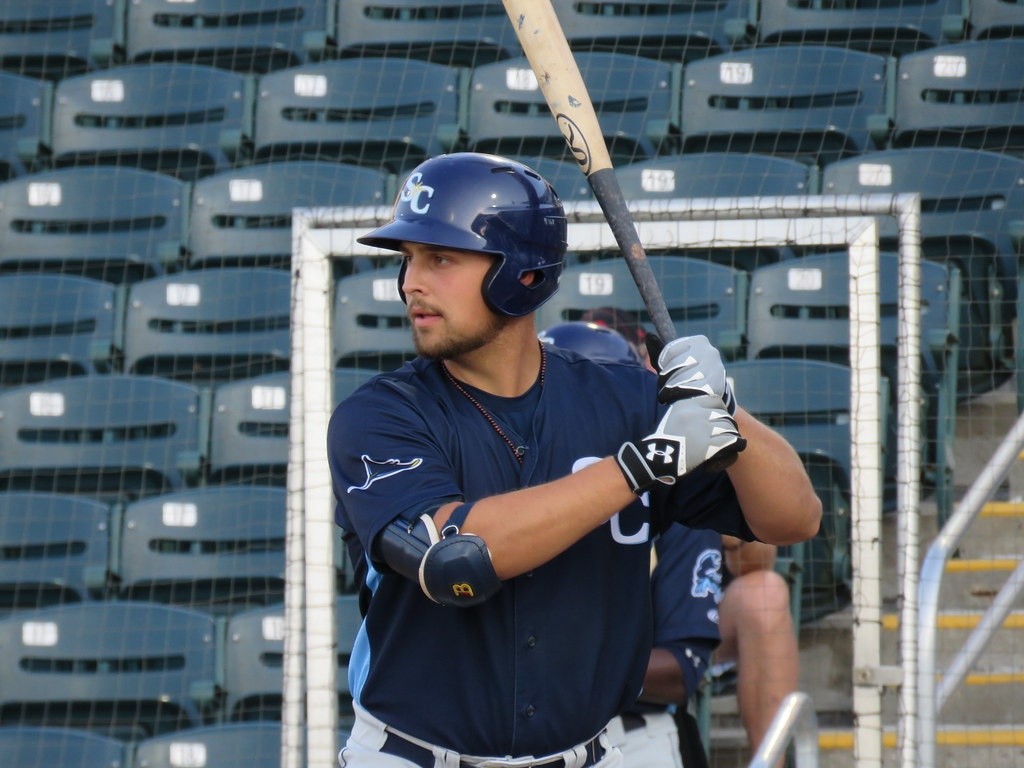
441,337,547,468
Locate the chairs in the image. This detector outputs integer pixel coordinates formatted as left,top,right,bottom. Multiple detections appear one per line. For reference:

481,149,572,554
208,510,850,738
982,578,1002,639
0,2,1024,767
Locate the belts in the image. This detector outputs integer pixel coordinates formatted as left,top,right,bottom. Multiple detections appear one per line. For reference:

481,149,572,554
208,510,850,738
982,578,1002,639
380,728,608,768
620,713,647,733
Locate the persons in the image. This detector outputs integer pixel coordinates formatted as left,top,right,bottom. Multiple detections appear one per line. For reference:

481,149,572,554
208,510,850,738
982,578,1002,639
326,153,822,768
580,301,798,768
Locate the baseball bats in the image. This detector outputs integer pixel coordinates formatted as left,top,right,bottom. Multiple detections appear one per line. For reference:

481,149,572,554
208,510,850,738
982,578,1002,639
497,0,683,350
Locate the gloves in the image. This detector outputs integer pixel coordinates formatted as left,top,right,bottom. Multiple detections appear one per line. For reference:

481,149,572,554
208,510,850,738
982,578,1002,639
614,395,747,496
644,331,737,422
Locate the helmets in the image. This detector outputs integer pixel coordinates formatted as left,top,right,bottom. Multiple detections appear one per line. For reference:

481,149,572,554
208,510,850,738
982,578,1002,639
537,321,646,367
356,154,567,317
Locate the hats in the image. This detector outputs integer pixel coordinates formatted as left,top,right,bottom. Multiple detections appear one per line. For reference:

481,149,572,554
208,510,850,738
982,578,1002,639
579,305,645,346
